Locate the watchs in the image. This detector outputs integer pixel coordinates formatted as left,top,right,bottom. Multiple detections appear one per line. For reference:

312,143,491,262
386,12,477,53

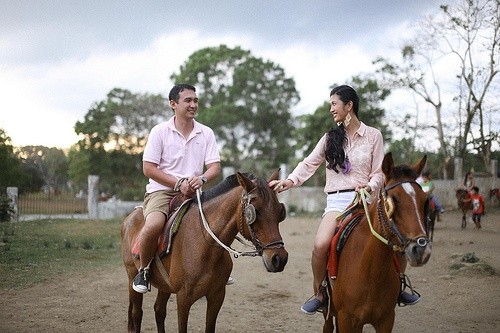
200,175,207,184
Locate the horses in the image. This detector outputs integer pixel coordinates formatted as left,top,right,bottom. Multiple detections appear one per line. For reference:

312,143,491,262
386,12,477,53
424,187,500,242
120,166,289,333
312,150,432,333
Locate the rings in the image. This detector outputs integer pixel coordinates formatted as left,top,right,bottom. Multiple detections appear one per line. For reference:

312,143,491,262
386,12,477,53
278,184,280,188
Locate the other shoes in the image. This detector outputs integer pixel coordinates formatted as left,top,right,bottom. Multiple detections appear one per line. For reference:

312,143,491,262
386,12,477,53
441,208,444,213
301,298,322,315
399,291,422,305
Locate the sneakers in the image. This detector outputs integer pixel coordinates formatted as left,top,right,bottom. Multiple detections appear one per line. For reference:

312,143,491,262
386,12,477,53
226,277,234,285
132,266,151,294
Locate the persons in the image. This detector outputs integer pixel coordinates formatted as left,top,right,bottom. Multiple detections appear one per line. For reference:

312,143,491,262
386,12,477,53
462,172,475,195
132,83,234,294
268,84,421,315
415,169,443,222
462,186,486,232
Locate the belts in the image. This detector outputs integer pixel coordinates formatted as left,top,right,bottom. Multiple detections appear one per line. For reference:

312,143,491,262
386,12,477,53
328,189,355,194
